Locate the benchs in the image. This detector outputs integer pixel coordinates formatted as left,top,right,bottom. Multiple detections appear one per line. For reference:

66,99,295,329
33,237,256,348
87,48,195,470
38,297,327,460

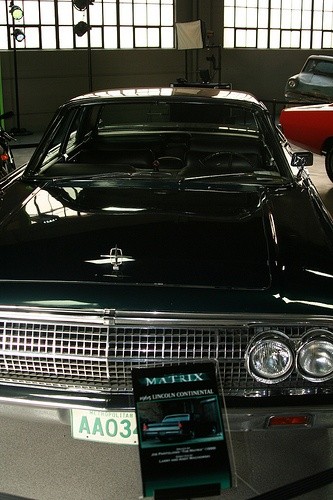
79,137,262,168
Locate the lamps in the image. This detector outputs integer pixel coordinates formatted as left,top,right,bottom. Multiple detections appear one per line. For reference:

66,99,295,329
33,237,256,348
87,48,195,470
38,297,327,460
74,0,95,9
73,21,92,37
13,29,25,42
11,6,24,20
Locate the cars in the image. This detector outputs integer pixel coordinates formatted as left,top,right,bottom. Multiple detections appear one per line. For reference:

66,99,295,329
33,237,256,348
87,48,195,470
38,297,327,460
285,55,333,107
1,88,333,446
280,94,333,183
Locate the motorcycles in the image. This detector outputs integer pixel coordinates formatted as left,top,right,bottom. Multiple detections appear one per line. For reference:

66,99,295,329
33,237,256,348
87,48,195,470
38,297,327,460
0,111,17,179
